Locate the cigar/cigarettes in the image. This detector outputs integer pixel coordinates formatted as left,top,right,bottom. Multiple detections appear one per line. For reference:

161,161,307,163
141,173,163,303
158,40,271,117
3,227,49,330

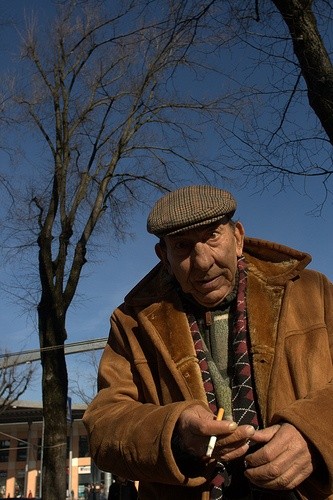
206,407,225,457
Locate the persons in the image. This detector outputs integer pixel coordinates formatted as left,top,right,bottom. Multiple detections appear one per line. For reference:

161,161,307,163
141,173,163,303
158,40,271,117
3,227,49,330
82,186,333,499
107,472,138,498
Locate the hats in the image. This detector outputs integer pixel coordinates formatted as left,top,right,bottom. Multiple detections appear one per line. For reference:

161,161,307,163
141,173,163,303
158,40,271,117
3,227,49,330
147,185,236,240
